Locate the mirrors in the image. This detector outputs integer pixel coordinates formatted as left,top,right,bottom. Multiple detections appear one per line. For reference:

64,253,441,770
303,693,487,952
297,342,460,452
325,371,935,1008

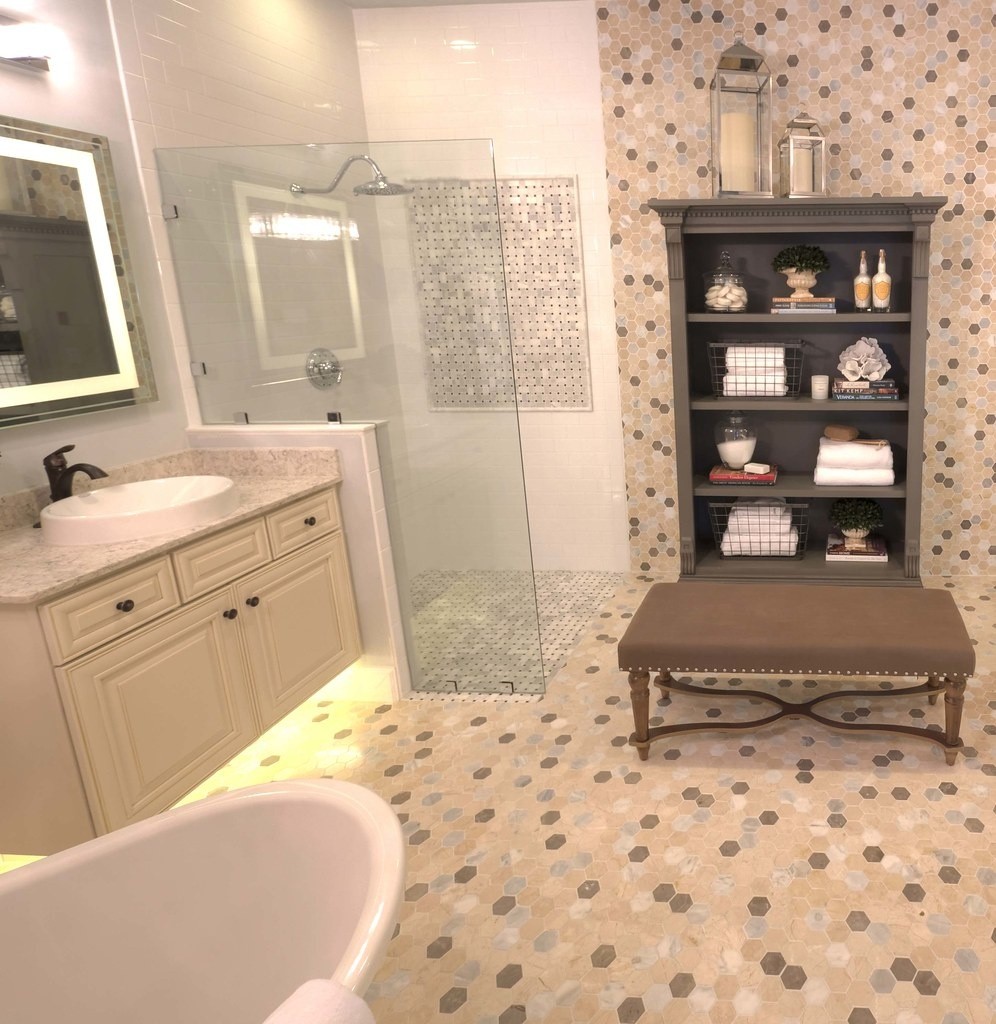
0,115,158,429
234,181,364,365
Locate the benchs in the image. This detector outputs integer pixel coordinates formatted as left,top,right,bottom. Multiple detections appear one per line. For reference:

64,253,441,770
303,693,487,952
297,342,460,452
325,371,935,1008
618,584,975,766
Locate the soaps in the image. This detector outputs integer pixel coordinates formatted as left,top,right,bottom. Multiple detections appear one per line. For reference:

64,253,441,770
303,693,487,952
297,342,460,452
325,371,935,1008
744,461,771,476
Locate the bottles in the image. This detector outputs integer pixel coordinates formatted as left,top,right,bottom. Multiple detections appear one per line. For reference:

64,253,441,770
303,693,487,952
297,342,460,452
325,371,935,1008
853,249,872,312
714,411,758,471
811,374,829,399
872,249,891,312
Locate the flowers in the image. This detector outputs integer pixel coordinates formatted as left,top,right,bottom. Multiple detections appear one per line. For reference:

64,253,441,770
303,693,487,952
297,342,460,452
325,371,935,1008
838,337,891,381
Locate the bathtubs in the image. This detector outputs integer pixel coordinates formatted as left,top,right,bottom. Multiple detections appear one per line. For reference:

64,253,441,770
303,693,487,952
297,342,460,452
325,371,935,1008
0,771,410,1024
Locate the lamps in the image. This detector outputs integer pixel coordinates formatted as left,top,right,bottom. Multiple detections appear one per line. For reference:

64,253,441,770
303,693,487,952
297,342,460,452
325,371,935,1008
1,9,50,74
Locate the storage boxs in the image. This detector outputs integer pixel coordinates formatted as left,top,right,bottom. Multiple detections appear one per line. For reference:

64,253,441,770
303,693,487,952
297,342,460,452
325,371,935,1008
707,337,806,399
706,497,808,560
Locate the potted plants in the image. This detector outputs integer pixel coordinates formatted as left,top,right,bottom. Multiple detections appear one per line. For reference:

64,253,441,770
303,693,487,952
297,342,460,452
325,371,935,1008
829,498,884,538
772,245,828,298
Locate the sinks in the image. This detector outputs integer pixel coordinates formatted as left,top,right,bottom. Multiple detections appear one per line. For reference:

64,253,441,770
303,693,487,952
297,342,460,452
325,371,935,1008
37,474,239,545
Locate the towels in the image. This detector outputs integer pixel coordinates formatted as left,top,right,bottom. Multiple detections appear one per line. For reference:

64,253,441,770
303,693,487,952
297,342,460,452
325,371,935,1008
816,435,894,470
722,374,789,397
261,977,379,1024
724,346,786,375
813,462,896,487
719,496,802,558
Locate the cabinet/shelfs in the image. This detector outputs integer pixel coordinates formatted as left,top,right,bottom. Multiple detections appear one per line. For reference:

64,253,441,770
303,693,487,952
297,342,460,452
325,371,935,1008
646,197,946,582
0,474,366,856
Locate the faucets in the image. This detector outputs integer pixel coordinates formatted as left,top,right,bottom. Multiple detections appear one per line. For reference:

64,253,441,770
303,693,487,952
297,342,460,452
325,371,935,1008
43,443,112,502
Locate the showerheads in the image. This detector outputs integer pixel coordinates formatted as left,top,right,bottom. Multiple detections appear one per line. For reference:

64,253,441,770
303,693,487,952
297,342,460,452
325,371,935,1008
351,176,417,198
